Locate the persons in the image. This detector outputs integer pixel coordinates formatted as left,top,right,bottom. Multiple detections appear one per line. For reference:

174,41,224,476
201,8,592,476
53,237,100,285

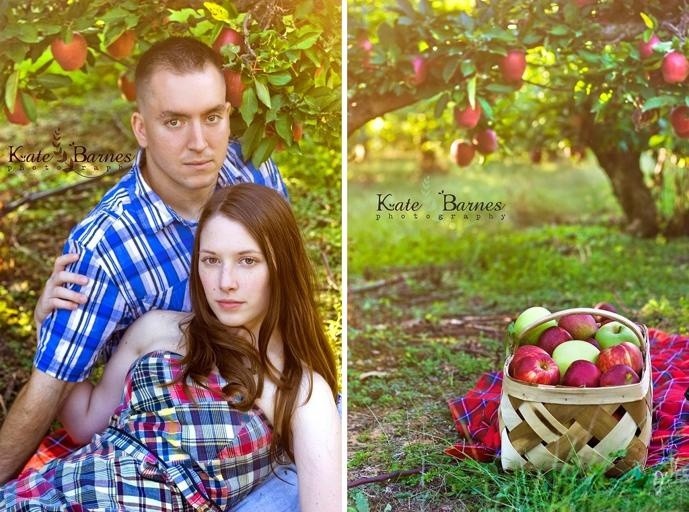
0,36,302,512
0,178,340,512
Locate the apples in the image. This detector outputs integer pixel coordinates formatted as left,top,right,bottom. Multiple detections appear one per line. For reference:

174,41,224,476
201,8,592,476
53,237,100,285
509,303,643,445
4,0,689,167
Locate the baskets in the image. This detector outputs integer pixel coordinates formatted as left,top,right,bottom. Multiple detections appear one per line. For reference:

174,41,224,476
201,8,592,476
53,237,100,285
497,308,653,481
